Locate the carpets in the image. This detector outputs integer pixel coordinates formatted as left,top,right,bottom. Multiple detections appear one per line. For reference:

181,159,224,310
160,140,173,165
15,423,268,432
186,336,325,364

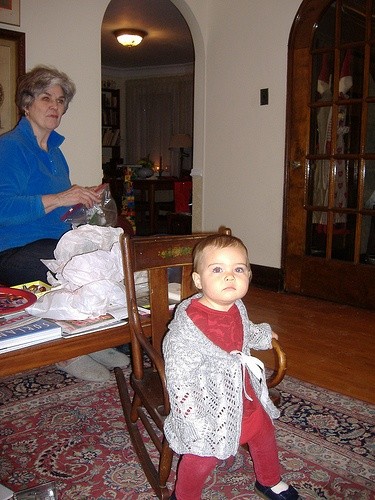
0,332,375,500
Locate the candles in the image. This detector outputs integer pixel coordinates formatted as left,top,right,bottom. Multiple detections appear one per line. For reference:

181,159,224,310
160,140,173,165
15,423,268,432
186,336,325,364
165,166,168,169
156,166,160,170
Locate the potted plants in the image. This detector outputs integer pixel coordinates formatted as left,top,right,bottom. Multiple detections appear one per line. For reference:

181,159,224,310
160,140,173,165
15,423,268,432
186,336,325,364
134,154,155,178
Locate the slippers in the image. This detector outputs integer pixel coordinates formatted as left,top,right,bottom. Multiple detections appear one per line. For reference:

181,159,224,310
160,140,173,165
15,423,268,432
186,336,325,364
88,348,130,371
56,355,110,383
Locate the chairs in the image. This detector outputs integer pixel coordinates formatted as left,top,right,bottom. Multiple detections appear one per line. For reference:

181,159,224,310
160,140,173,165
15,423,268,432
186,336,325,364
109,229,286,500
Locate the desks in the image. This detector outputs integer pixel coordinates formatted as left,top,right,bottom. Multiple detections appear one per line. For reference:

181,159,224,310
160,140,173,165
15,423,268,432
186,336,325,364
103,174,180,235
0,274,184,376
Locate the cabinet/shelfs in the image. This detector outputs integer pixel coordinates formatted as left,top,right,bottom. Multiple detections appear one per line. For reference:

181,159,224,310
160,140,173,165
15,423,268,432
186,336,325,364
283,0,375,311
101,87,121,159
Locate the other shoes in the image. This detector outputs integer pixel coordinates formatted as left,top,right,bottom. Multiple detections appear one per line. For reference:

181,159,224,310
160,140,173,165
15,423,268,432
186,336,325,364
254,480,299,500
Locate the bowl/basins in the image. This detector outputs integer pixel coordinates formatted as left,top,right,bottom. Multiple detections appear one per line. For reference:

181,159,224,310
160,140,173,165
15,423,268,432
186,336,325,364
10,280,51,296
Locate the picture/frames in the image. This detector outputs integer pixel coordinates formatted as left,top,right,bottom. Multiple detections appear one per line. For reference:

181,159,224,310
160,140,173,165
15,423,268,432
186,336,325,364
0,27,26,136
0,0,21,28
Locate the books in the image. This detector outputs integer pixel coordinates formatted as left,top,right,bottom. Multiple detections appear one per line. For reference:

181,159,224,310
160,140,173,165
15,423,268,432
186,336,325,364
0,282,181,354
102,94,120,163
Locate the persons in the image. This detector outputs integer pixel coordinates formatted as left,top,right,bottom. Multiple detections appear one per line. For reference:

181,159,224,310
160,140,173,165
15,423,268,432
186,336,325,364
0,67,129,383
162,233,299,500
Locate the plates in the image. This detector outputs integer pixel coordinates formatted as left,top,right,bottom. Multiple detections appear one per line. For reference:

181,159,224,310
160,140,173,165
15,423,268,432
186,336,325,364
0,287,37,315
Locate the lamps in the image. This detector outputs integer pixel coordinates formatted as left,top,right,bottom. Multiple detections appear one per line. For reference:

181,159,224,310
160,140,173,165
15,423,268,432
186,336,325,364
167,132,193,179
113,28,148,47
